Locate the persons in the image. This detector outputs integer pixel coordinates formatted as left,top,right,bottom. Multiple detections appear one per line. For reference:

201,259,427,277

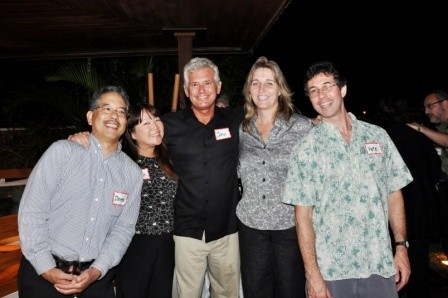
237,58,318,298
17,84,144,298
406,92,448,175
116,99,179,298
280,58,414,298
164,57,240,298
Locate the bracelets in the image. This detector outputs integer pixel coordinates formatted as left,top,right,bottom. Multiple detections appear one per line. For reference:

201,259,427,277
416,123,426,133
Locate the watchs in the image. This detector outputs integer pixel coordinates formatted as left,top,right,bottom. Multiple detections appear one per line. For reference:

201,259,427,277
394,240,410,248
